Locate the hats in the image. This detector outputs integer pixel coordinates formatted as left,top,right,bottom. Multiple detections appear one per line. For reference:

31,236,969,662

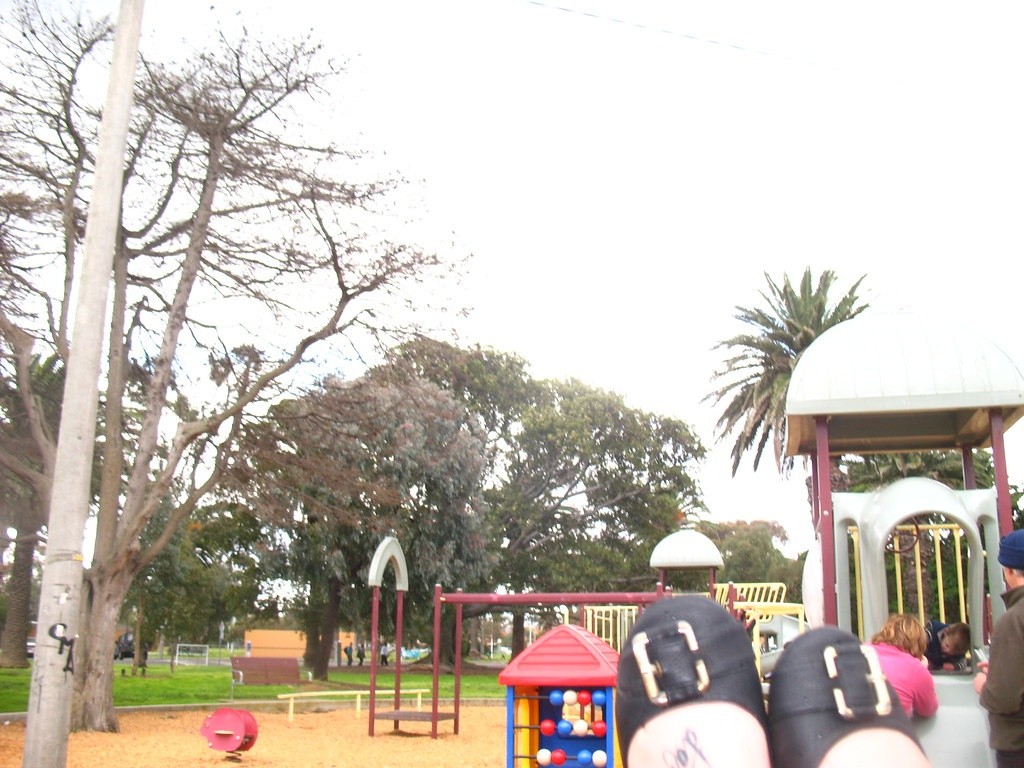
998,529,1024,568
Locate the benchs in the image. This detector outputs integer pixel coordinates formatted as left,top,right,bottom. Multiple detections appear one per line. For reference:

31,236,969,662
230,656,313,699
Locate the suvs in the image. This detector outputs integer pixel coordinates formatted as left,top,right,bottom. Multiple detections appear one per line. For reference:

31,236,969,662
114,631,147,660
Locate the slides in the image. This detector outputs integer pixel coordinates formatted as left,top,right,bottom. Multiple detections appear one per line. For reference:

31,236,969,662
748,611,816,678
909,671,1000,768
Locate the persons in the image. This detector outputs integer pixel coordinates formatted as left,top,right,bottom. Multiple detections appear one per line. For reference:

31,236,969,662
358,644,366,666
865,614,938,721
381,643,388,666
972,528,1024,768
344,642,353,665
924,620,971,670
